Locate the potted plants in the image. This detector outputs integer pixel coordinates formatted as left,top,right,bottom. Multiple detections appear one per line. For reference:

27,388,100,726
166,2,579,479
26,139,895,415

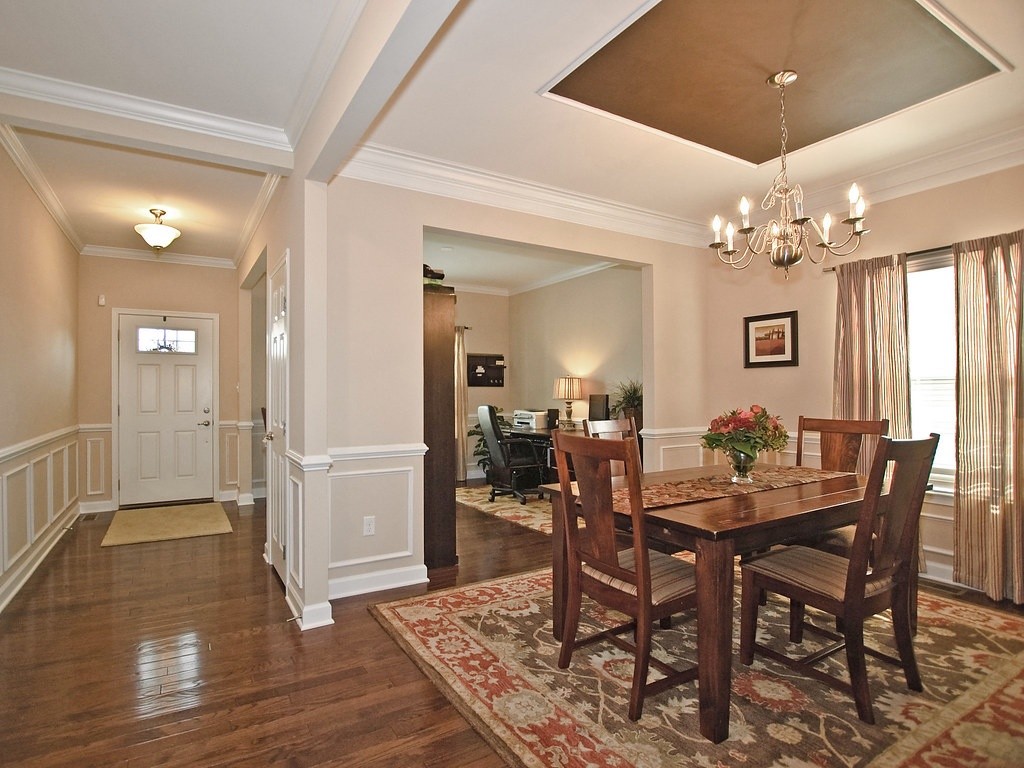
467,406,513,484
611,377,643,436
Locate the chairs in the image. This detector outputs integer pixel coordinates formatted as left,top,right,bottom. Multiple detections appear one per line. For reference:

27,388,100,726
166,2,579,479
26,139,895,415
549,428,699,721
738,431,942,724
758,416,890,632
477,405,547,505
583,417,689,631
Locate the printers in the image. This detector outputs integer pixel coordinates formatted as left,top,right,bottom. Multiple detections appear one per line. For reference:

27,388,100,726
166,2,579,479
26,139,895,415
513,409,559,429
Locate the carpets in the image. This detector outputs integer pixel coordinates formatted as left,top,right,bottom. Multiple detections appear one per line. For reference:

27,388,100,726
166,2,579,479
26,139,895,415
100,502,234,548
455,484,586,538
367,546,1024,768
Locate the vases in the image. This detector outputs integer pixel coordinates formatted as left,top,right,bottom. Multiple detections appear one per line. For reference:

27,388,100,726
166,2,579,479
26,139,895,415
726,448,759,485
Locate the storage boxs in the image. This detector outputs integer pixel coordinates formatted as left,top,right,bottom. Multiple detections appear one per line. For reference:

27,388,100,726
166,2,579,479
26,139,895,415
496,360,505,365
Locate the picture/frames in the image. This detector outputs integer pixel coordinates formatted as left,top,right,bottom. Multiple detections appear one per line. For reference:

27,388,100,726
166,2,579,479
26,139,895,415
743,310,799,368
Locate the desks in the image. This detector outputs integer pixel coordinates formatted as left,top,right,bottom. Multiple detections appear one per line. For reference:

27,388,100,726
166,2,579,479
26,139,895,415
537,463,934,744
501,426,585,503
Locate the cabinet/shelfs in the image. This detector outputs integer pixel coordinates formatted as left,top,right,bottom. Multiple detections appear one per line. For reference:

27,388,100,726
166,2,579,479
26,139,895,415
466,353,507,388
423,292,460,570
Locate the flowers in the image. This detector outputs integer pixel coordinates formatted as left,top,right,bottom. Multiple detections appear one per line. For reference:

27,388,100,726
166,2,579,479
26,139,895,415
698,404,790,459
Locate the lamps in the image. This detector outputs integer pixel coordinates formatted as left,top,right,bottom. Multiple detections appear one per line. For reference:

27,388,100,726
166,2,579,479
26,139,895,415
553,374,584,431
708,70,872,280
134,209,182,250
